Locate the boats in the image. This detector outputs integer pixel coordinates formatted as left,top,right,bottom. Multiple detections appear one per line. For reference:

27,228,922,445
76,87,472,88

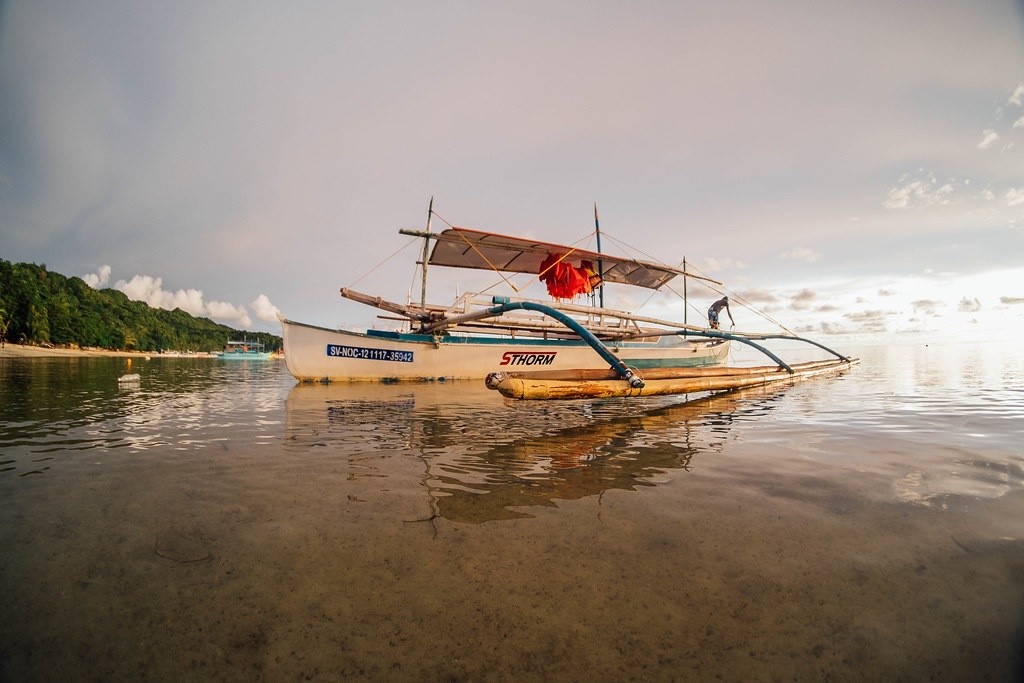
216,340,271,361
272,196,734,379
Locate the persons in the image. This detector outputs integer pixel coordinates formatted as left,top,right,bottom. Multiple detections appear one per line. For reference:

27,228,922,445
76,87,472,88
708,297,735,343
